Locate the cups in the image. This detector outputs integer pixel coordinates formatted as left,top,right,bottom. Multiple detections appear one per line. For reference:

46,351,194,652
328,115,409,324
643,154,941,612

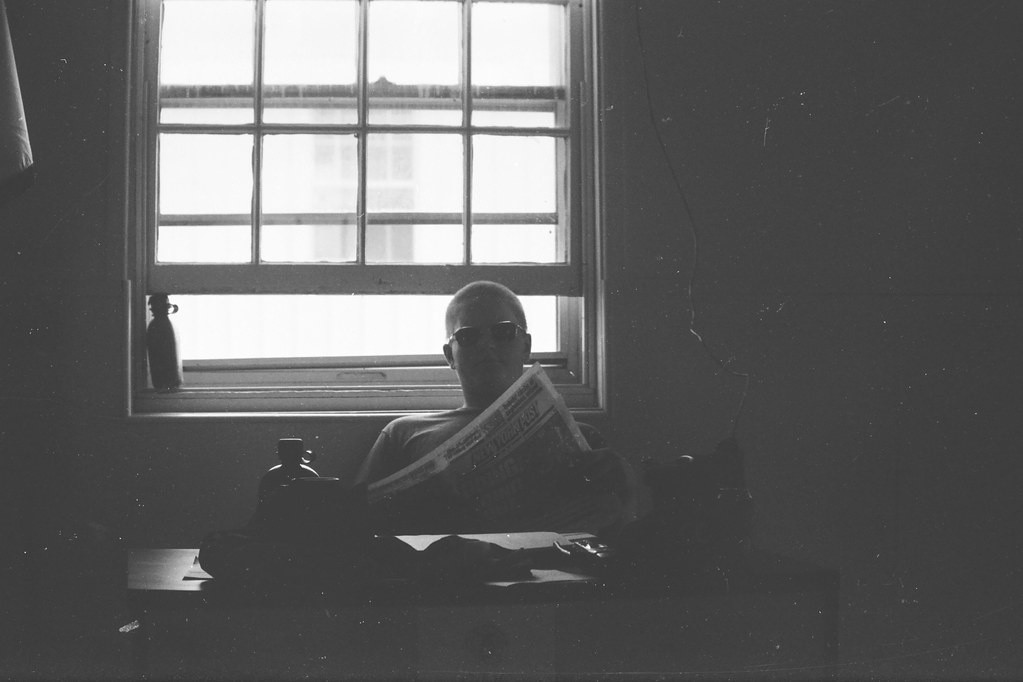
292,476,340,530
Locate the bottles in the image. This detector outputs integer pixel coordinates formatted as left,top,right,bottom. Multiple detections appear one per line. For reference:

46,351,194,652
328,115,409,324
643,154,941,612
258,435,312,531
144,294,184,393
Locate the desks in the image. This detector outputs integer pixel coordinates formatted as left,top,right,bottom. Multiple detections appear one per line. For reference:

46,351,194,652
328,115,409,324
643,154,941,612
124,544,609,682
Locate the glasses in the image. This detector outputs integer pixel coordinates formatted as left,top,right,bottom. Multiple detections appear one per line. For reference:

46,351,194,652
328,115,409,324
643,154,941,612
448,320,527,347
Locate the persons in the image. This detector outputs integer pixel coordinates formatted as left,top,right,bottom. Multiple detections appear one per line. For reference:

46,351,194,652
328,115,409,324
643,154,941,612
352,278,612,494
